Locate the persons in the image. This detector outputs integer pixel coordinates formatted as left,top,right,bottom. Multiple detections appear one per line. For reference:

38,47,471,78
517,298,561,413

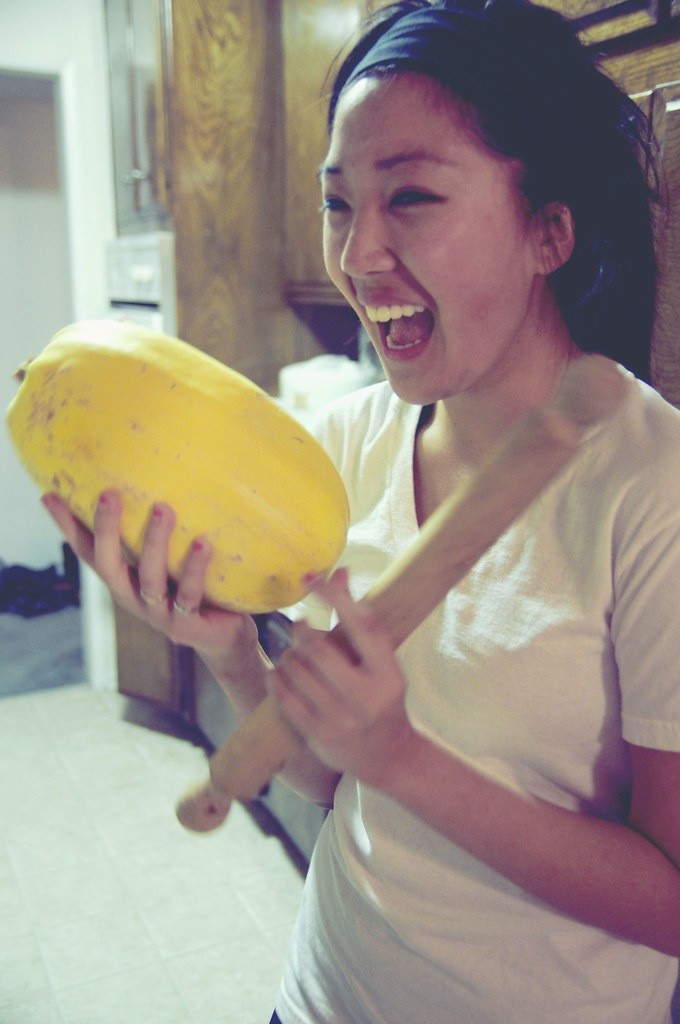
40,0,680,1024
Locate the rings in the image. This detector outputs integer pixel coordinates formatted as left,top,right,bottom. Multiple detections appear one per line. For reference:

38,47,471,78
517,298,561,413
141,591,168,603
173,602,198,613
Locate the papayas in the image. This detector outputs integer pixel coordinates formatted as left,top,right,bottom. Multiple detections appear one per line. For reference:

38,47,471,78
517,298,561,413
9,320,351,614
